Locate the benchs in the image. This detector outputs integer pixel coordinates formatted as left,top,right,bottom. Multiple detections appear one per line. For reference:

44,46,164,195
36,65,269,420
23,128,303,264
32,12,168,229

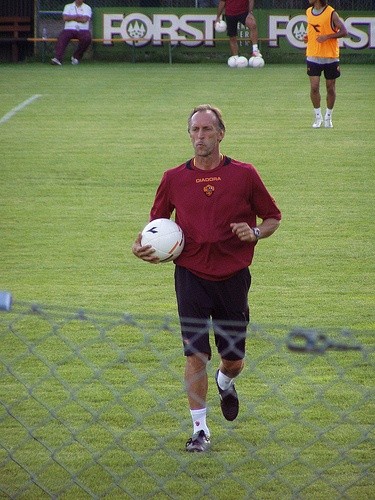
27,38,277,64
0,15,31,63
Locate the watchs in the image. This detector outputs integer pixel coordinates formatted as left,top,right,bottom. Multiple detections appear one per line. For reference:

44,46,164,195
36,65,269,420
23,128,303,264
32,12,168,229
252,226,261,239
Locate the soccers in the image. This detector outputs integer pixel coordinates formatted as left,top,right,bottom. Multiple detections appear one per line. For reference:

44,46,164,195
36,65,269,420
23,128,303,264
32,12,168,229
252,57,264,68
236,56,248,68
140,218,185,263
228,55,238,68
215,21,227,33
248,56,255,67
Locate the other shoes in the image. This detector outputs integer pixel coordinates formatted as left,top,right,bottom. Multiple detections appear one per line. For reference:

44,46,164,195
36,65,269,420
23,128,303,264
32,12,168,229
71,57,79,64
252,50,262,57
49,57,61,65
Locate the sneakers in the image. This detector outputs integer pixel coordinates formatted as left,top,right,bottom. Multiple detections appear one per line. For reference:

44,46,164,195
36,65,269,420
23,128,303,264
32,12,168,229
325,113,333,127
215,369,239,421
312,117,323,127
184,430,210,452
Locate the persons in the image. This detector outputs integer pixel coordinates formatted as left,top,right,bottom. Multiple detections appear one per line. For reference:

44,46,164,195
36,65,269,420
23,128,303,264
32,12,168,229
51,0,93,65
133,105,281,453
303,0,348,128
216,0,263,60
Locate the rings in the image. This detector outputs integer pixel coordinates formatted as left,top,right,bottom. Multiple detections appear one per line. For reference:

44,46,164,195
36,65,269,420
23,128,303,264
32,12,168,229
242,232,245,236
